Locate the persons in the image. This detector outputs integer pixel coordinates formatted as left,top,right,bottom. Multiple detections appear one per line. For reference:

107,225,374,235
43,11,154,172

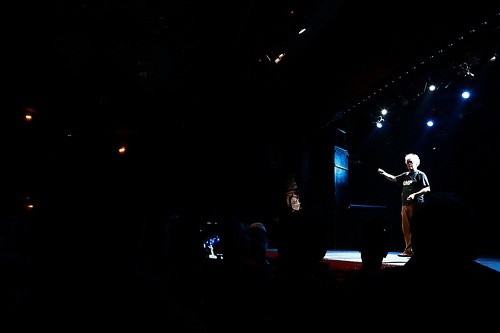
377,152,431,257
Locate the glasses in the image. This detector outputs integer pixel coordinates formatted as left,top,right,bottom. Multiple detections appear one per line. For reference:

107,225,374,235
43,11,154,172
407,161,412,163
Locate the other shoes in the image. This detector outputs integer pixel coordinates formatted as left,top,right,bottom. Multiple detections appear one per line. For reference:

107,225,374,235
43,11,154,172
398,250,413,257
